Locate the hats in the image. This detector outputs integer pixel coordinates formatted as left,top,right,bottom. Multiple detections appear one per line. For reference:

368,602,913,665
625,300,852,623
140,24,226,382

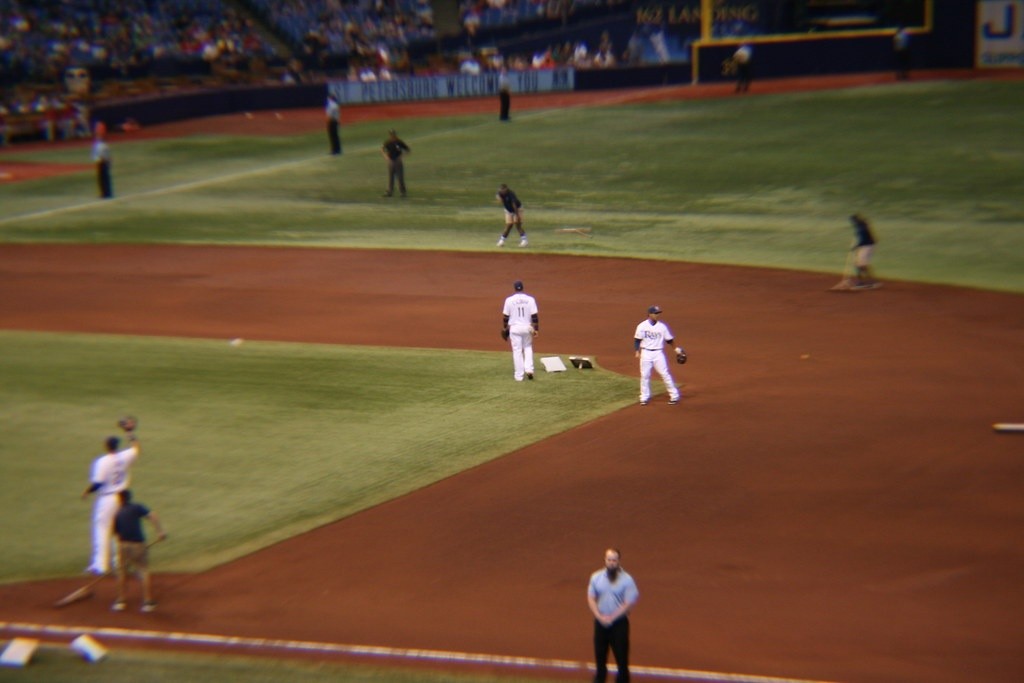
515,281,522,289
648,306,662,313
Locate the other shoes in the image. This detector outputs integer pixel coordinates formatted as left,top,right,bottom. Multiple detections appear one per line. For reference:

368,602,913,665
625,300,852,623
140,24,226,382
668,398,678,404
640,400,648,405
142,602,158,612
497,243,504,247
518,242,528,247
112,602,127,610
527,373,533,380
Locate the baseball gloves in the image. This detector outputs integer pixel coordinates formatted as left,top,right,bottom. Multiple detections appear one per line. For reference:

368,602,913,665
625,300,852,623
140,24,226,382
501,328,509,341
117,417,137,431
675,349,687,364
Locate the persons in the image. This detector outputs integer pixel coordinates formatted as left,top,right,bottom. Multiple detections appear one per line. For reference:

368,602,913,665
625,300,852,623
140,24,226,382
108,489,166,613
587,547,639,683
895,25,908,80
848,214,884,291
734,41,751,95
634,306,687,405
501,280,539,382
0,0,760,115
326,94,342,155
498,67,512,122
496,184,528,247
381,128,411,197
92,136,113,199
79,416,141,574
